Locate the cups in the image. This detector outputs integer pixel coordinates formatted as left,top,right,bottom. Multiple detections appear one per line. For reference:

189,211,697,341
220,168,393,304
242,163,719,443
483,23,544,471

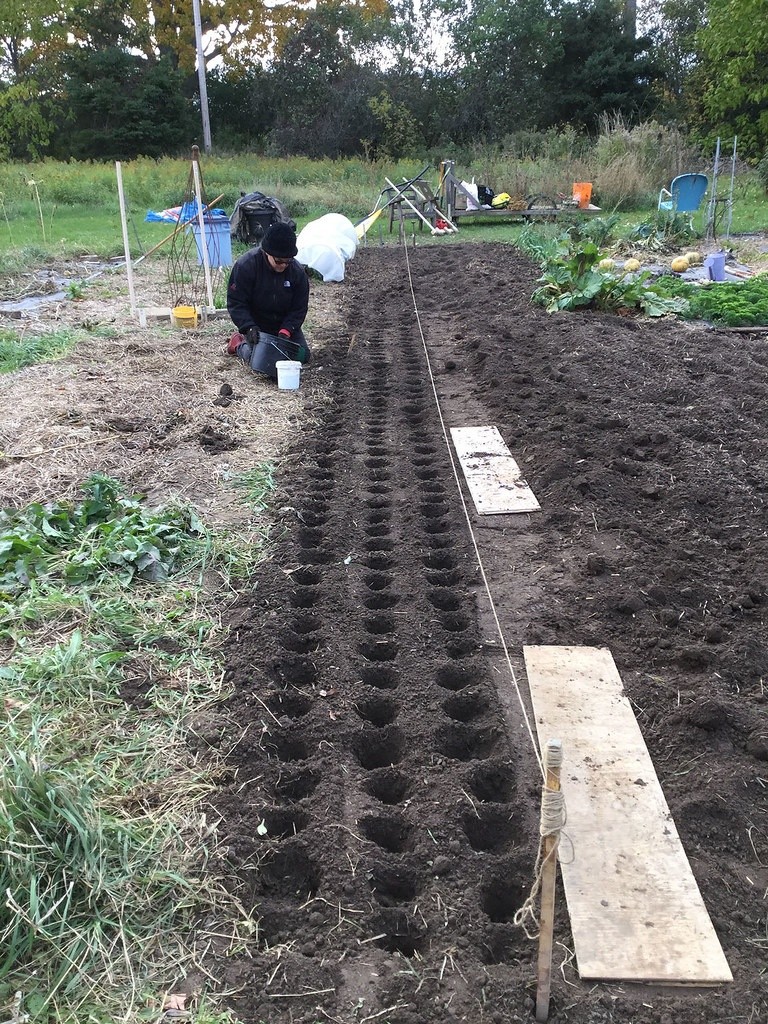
275,360,303,389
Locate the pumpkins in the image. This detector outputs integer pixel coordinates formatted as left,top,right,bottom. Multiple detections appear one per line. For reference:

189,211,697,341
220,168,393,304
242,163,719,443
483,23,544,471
601,252,699,272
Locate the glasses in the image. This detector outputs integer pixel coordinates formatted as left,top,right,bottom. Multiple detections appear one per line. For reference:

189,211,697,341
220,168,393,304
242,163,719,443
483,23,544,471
273,256,293,265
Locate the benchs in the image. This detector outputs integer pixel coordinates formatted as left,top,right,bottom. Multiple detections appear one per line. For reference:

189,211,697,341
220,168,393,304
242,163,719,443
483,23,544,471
384,183,438,231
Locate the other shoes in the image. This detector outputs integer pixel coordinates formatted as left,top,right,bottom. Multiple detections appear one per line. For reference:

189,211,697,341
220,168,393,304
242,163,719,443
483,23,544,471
228,333,244,354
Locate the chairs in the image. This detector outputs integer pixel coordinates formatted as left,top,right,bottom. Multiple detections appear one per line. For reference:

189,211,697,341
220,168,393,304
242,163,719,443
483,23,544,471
658,172,709,230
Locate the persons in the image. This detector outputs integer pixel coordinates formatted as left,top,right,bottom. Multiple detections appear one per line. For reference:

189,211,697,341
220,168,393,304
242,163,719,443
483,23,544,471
227,222,311,363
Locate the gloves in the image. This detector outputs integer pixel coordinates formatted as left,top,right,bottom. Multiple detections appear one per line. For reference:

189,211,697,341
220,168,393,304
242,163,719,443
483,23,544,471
246,326,261,344
279,333,292,342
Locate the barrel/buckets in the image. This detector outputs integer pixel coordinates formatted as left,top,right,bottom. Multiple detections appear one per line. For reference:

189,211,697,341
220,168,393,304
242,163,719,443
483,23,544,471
192,214,233,269
173,294,197,332
251,331,301,378
572,181,592,208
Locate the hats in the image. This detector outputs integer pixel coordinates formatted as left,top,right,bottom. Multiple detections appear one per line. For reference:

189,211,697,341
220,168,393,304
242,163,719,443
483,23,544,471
262,222,298,258
